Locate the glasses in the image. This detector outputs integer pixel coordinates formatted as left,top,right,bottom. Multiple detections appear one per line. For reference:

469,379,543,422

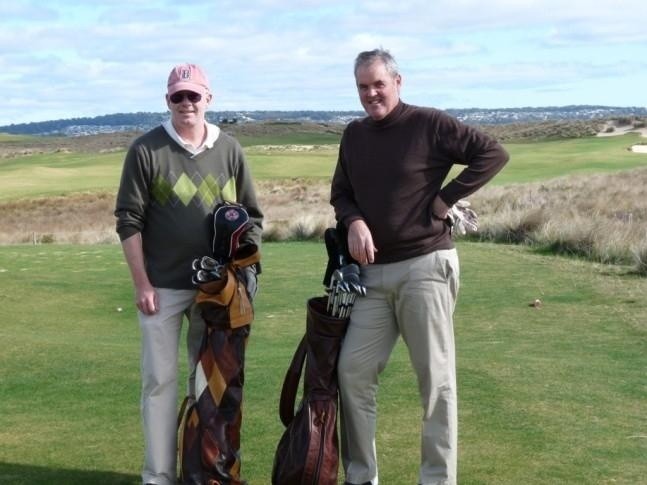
168,92,202,103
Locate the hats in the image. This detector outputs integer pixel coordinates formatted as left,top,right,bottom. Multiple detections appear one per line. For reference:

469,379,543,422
167,63,209,96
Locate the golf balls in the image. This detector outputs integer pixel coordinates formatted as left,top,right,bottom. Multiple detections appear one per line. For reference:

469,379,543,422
117,308,123,313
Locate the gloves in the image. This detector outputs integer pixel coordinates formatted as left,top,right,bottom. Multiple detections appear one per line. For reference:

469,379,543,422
447,199,480,237
234,262,261,304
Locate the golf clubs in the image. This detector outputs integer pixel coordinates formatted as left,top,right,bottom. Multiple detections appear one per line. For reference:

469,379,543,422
323,227,366,318
191,199,260,293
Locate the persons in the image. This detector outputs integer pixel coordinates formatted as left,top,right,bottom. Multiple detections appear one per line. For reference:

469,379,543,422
329,48,509,484
110,61,264,484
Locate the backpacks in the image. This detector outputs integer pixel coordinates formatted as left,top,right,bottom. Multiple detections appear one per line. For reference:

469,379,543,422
175,243,265,485
269,294,351,485
